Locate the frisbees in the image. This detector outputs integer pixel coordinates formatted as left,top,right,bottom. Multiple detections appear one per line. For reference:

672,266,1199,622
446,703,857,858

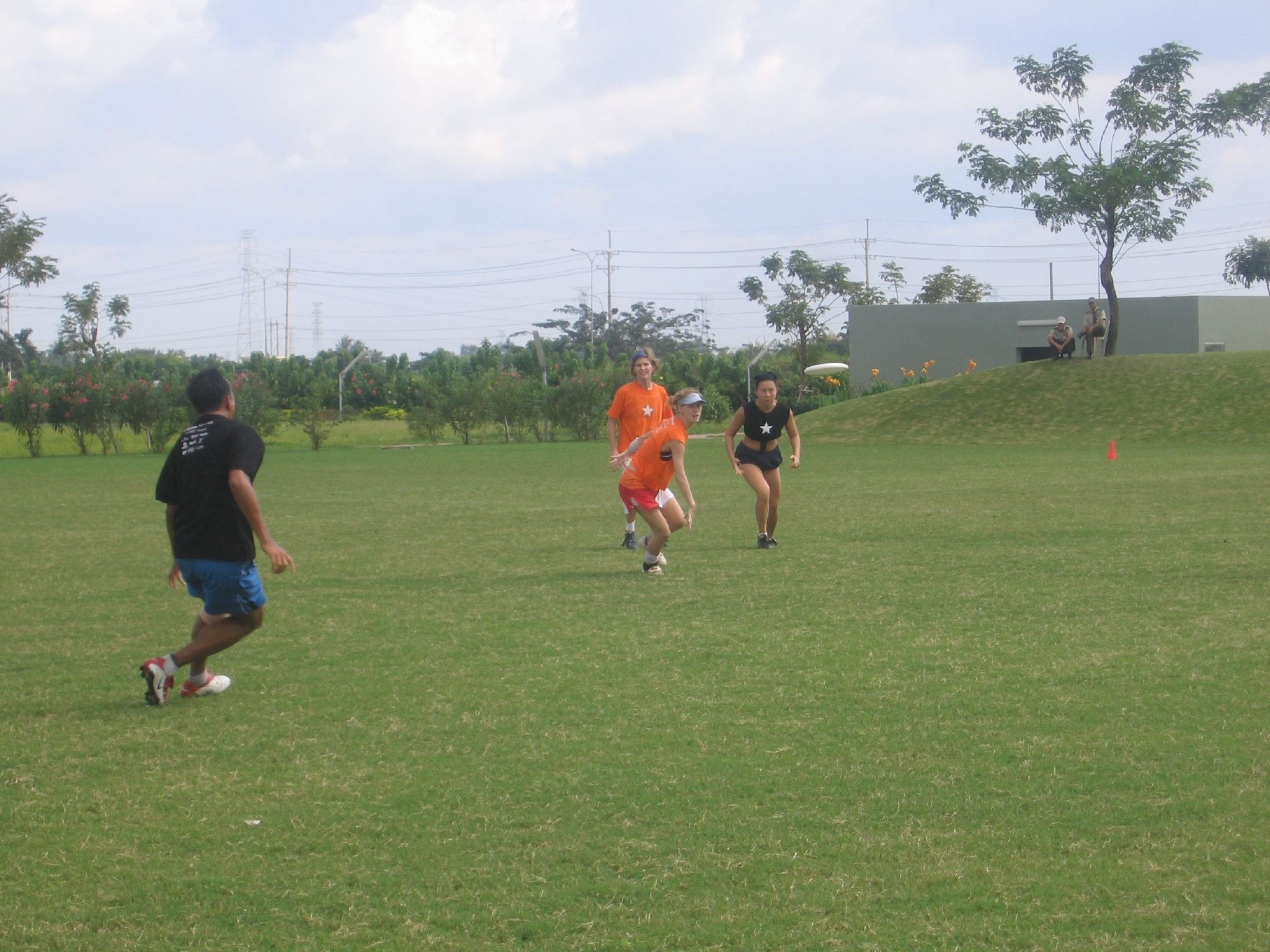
804,362,849,375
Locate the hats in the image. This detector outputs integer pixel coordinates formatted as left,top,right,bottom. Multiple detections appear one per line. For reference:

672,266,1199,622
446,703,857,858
1087,297,1095,303
677,392,706,406
1056,316,1066,324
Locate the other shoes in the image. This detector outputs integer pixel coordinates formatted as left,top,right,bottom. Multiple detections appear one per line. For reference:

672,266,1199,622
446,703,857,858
1068,352,1072,359
769,536,778,549
1053,355,1059,360
758,532,769,549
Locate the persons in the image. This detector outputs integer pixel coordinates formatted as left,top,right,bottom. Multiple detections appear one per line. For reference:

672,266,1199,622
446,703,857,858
139,368,297,705
1047,316,1076,360
606,347,687,551
1077,297,1107,360
724,371,801,549
609,386,706,575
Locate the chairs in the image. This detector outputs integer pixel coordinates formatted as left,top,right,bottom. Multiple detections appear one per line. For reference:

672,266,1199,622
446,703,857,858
1080,319,1110,357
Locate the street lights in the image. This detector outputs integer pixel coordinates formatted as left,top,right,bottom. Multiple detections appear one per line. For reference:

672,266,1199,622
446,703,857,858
243,267,287,356
571,248,620,360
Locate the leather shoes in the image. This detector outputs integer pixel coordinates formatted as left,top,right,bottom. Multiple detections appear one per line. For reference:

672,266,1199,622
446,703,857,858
1088,353,1094,359
1077,331,1083,338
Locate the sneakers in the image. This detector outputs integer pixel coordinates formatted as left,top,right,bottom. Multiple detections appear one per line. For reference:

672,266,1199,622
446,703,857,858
625,529,639,549
181,673,231,697
642,560,664,576
639,536,667,566
139,657,174,705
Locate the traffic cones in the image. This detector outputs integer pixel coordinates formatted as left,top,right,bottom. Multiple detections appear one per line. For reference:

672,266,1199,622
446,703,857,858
1108,441,1117,459
